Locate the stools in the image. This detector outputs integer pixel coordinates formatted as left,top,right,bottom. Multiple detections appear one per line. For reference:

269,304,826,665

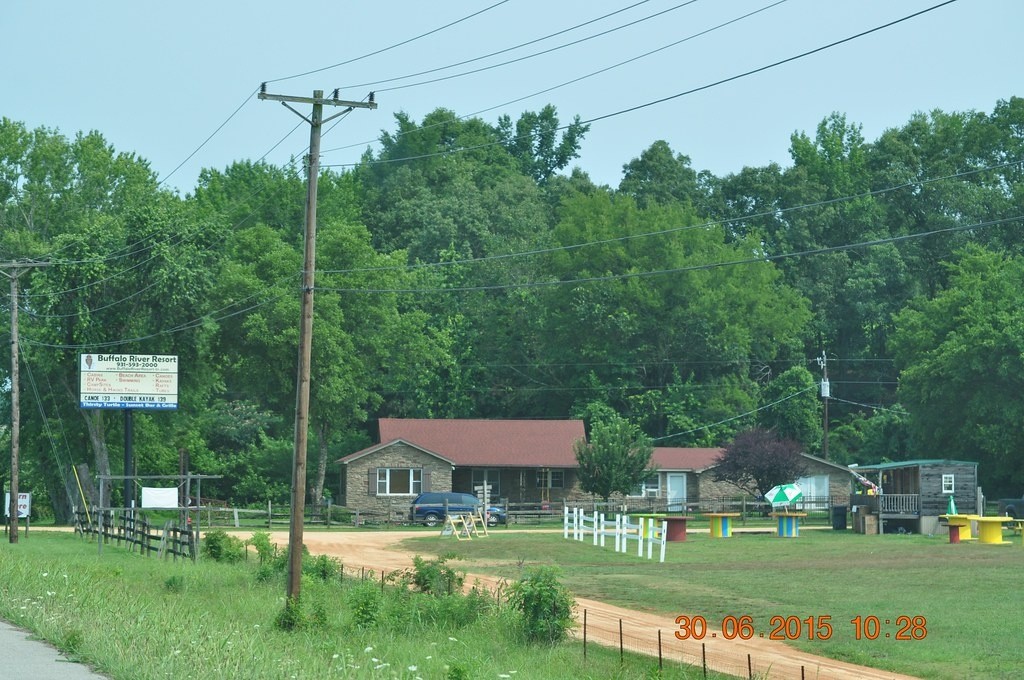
941,524,966,544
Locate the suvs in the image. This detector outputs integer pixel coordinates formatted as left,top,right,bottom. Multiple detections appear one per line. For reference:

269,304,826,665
408,491,506,527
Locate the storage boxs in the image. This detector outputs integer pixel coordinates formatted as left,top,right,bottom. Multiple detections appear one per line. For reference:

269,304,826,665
854,505,878,535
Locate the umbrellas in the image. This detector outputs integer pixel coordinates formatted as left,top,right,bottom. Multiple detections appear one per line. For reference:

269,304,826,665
764,484,803,510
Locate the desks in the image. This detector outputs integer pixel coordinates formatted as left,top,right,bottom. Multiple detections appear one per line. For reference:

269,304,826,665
967,517,1013,545
768,513,807,537
1010,519,1024,546
702,513,740,537
627,514,666,538
940,515,979,540
657,516,695,541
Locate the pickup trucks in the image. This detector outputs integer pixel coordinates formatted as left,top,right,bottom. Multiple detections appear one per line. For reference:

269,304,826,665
999,494,1024,526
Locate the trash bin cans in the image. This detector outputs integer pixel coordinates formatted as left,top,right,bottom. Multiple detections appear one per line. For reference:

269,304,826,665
832,506,847,530
